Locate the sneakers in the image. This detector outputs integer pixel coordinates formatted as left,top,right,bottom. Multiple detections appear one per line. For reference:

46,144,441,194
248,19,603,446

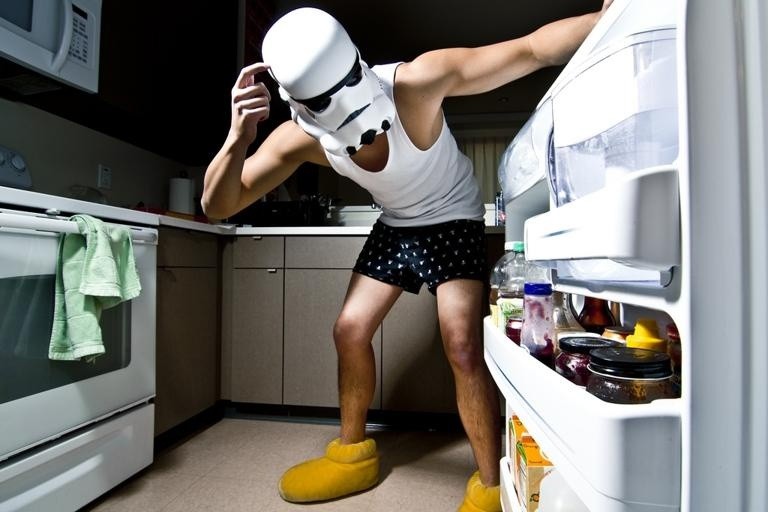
457,470,502,512
278,437,380,504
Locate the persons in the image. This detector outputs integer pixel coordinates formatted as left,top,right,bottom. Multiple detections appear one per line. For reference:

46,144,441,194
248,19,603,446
199,1,619,512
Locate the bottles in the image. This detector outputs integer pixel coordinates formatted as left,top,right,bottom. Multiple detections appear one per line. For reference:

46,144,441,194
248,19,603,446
518,283,558,357
486,240,514,330
574,297,616,334
498,241,553,345
555,316,680,403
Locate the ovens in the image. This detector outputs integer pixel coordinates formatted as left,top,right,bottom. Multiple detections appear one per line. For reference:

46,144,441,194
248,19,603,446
0,207,162,512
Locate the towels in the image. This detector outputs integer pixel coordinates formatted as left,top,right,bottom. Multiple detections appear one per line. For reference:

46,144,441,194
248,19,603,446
42,213,142,367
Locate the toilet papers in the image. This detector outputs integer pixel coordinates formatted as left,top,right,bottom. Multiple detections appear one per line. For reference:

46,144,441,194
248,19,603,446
167,176,197,217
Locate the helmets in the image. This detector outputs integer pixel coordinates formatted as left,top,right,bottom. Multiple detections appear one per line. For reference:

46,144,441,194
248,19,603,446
261,7,397,157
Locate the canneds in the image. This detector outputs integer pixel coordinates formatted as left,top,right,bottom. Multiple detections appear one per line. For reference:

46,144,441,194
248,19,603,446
666,322,681,397
586,347,671,405
555,336,623,390
601,326,635,346
507,316,522,346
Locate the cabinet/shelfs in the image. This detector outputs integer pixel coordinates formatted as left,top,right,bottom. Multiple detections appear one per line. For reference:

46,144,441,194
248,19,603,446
158,225,222,438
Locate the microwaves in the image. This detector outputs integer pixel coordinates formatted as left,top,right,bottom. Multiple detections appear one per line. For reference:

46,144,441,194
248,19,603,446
0,1,104,97
